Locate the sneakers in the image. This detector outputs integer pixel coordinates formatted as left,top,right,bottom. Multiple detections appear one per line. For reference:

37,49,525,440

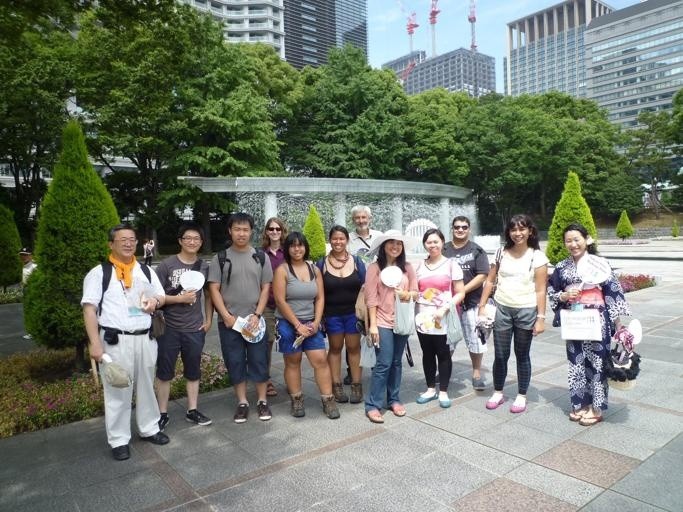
291,393,304,417
351,383,362,403
321,396,340,418
185,409,212,425
159,416,169,432
256,401,273,420
332,382,349,403
234,403,250,423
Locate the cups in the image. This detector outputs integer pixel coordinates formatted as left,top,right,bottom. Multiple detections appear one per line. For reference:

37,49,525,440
434,317,443,329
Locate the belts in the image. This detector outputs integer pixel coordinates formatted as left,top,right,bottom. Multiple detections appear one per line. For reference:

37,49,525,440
102,326,149,335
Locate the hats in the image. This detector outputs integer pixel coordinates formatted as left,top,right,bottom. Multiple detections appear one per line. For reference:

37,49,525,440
100,354,133,387
370,230,412,256
18,247,33,256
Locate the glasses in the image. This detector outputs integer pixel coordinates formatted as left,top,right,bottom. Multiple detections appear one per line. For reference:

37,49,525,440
454,226,469,230
268,227,281,231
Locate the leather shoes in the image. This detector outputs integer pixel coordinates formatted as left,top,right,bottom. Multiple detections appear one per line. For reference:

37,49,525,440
113,445,129,460
141,432,170,444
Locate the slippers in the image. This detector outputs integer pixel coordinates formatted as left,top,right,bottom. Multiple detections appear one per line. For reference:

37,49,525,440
569,410,603,426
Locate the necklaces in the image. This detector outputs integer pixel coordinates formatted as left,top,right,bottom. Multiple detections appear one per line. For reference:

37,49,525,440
328,250,349,269
424,260,441,271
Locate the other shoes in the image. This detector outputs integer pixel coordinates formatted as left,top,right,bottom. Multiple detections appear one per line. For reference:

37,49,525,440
472,376,485,389
438,394,452,408
344,375,352,385
434,376,439,384
486,395,504,409
416,393,438,403
510,400,526,413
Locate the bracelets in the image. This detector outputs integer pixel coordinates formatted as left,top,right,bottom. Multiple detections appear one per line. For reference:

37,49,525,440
536,315,546,319
253,312,261,319
295,322,300,329
477,304,485,308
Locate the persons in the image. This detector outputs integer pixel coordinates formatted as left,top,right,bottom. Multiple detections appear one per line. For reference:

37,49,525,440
80,225,170,461
548,224,632,426
477,213,548,415
428,216,488,391
316,226,367,405
142,238,155,266
413,230,465,408
255,218,290,397
153,224,213,432
17,247,44,340
208,213,274,424
271,232,341,420
343,205,386,385
364,230,419,425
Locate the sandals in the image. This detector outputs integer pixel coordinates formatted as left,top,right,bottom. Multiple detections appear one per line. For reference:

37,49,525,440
389,403,407,416
366,409,384,423
266,383,278,396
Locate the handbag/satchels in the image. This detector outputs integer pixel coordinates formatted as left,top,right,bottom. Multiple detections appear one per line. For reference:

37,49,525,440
152,309,166,338
146,249,151,256
607,350,641,392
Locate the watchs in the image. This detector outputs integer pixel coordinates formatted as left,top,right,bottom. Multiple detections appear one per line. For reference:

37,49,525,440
153,296,159,309
